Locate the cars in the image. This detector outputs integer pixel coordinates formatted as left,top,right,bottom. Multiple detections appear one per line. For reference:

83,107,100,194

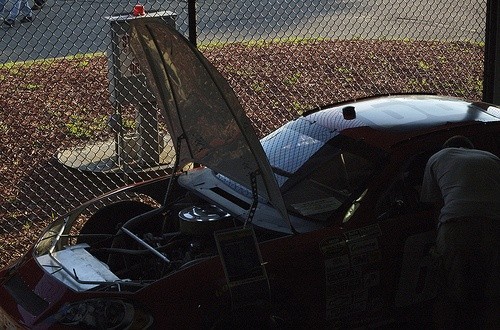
0,18,500,330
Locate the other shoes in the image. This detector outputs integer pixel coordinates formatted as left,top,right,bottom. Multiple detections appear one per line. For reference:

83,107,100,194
20,16,32,23
0,16,15,26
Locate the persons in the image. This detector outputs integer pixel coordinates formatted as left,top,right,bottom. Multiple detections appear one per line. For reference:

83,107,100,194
3,0,33,26
422,135,500,330
